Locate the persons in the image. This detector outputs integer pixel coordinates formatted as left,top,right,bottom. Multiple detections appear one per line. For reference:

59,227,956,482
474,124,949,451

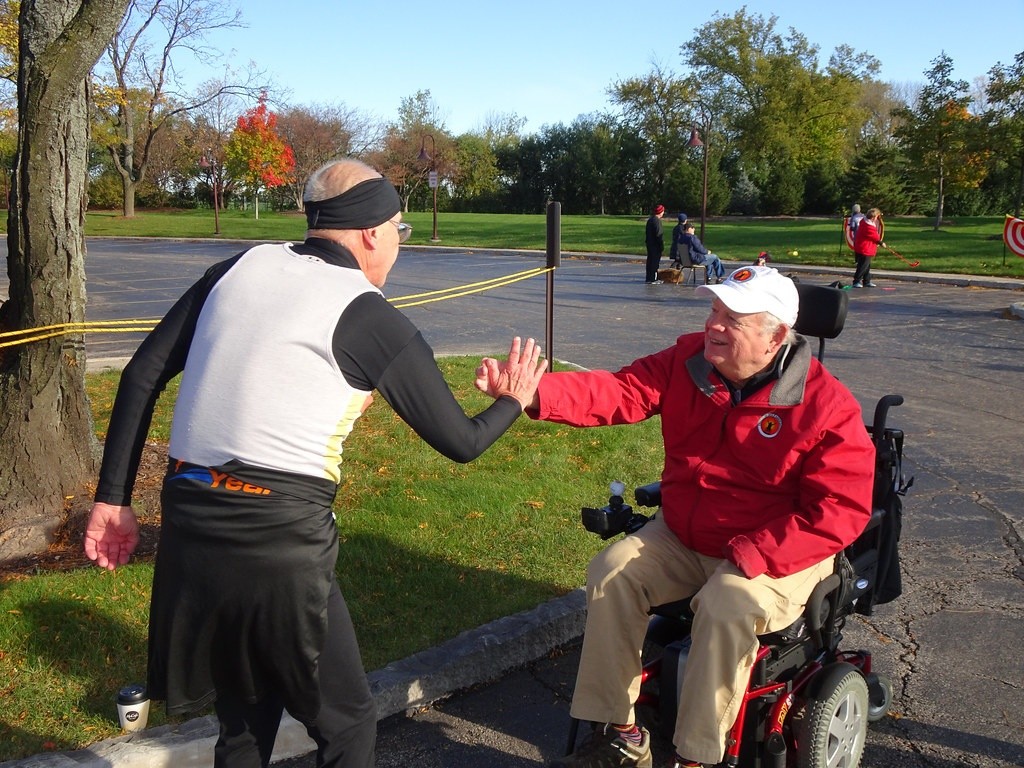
84,157,549,768
669,214,727,284
644,205,665,284
850,203,885,289
473,265,875,768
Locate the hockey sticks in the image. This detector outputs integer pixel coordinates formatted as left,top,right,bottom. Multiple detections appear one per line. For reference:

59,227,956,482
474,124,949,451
886,246,920,268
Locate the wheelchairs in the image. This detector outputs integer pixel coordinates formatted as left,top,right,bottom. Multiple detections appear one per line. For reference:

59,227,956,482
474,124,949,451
565,282,914,768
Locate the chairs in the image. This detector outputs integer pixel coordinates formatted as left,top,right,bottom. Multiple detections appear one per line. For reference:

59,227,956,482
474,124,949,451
670,239,706,286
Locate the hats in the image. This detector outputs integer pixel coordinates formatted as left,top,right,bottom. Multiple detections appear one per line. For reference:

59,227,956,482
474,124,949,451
694,265,800,328
682,223,694,230
677,213,688,222
654,204,665,214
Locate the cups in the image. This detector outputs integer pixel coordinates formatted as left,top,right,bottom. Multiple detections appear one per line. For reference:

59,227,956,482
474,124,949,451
117,684,150,733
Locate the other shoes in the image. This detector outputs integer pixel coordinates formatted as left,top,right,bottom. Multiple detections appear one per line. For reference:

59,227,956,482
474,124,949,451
646,280,664,284
716,280,723,284
707,279,713,285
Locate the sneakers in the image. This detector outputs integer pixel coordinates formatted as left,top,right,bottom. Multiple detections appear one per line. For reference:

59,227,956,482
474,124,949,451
863,282,876,287
661,756,704,768
853,282,863,288
543,725,653,768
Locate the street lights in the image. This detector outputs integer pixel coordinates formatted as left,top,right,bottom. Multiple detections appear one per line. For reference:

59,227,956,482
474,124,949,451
198,145,222,236
683,95,714,246
417,134,441,243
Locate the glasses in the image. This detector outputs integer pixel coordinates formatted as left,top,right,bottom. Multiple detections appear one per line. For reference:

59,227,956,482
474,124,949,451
690,227,694,229
388,219,413,244
